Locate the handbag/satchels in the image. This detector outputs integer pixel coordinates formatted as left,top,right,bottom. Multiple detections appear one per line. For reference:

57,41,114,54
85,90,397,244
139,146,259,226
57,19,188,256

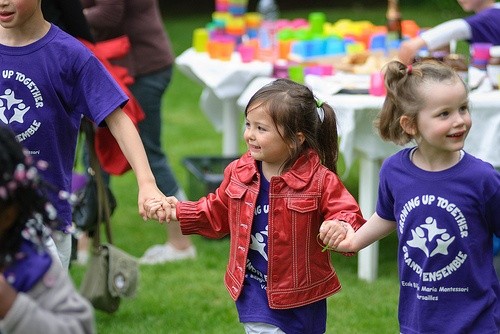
73,176,117,232
78,241,141,314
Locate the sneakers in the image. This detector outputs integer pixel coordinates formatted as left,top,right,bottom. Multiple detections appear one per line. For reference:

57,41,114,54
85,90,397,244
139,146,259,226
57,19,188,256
140,242,197,266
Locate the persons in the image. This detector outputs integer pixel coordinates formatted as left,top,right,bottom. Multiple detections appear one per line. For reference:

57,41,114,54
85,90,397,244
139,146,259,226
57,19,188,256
0,0,178,275
83,0,198,266
143,76,368,334
332,58,500,334
395,0,500,64
0,114,97,334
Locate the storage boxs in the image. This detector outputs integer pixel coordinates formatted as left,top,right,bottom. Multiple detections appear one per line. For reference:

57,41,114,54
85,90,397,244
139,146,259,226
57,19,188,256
180,154,239,201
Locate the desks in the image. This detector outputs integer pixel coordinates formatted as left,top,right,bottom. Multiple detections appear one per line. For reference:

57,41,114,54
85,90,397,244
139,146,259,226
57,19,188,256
175,50,500,280
